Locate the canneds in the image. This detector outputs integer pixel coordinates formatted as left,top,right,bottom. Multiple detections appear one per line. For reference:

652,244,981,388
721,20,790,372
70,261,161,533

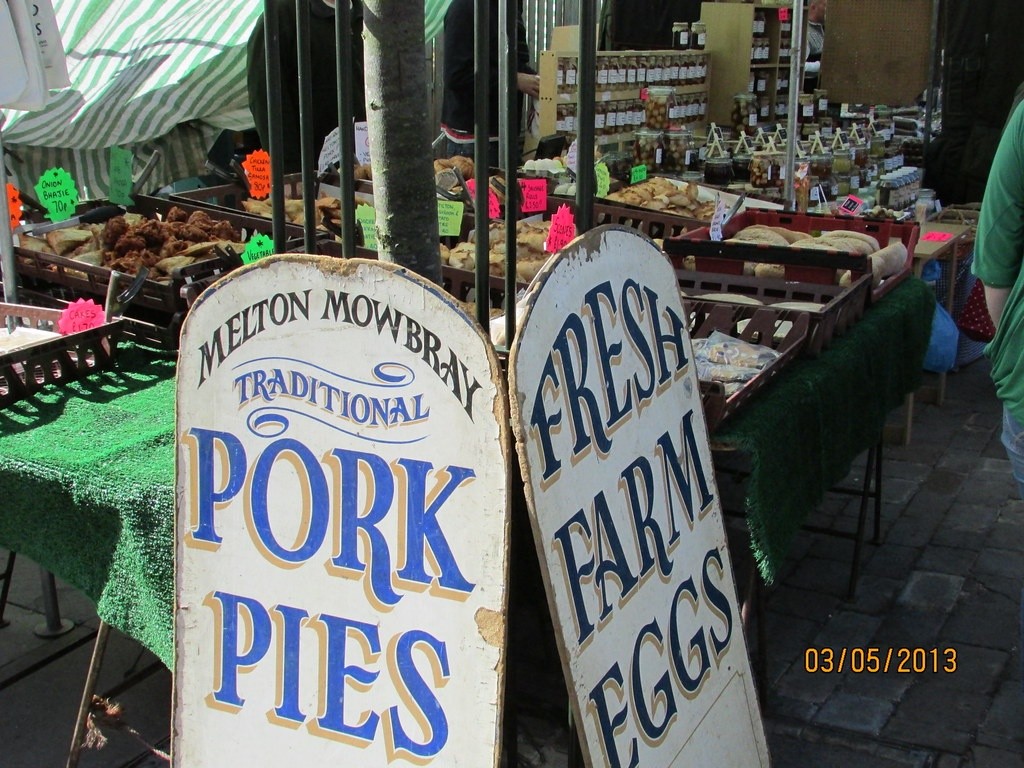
547,21,938,218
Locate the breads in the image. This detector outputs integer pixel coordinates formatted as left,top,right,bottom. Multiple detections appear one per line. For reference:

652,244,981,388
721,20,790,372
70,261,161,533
237,157,907,289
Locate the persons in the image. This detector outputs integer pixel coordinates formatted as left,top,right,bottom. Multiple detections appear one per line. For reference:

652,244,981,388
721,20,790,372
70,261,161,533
247,0,364,172
971,100,1024,502
441,0,540,173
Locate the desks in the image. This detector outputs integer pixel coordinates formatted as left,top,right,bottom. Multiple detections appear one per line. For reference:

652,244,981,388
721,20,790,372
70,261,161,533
709,275,939,724
936,241,988,366
902,221,973,447
0,338,178,767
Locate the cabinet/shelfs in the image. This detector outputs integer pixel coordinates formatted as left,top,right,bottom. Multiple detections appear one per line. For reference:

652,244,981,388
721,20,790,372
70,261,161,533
537,50,712,162
699,2,811,144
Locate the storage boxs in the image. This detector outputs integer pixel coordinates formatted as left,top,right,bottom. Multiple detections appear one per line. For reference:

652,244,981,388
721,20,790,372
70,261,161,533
179,240,532,311
1,195,336,349
663,209,921,303
681,299,810,437
666,253,873,358
941,201,982,212
0,302,127,411
169,170,378,251
459,194,711,242
593,175,793,238
437,164,504,213
924,209,980,261
540,174,747,239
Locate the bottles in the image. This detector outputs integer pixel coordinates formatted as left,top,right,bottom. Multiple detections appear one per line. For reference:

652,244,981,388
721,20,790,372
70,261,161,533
876,166,935,219
